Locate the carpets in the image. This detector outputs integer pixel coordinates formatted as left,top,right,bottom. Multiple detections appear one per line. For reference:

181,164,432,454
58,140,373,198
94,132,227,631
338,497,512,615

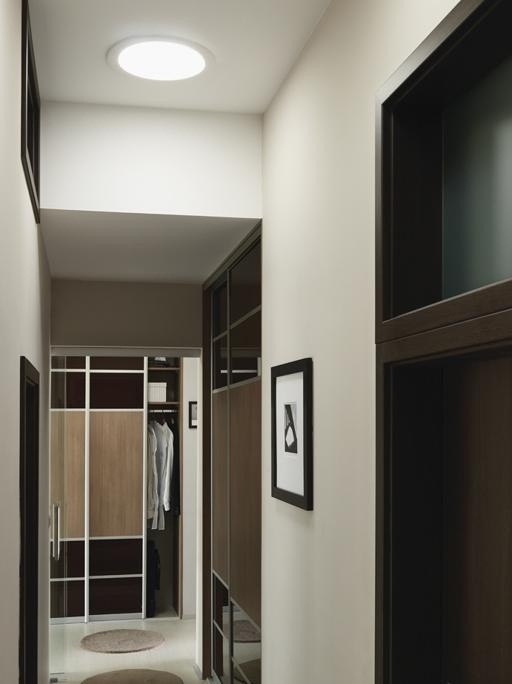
79,628,164,653
223,619,261,643
77,668,183,684
232,657,261,684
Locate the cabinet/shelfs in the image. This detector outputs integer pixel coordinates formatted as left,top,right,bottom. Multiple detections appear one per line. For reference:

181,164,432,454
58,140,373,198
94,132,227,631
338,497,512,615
50,354,184,622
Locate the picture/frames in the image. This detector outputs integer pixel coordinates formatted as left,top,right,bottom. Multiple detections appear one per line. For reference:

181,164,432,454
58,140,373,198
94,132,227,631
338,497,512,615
270,357,315,513
188,400,199,427
